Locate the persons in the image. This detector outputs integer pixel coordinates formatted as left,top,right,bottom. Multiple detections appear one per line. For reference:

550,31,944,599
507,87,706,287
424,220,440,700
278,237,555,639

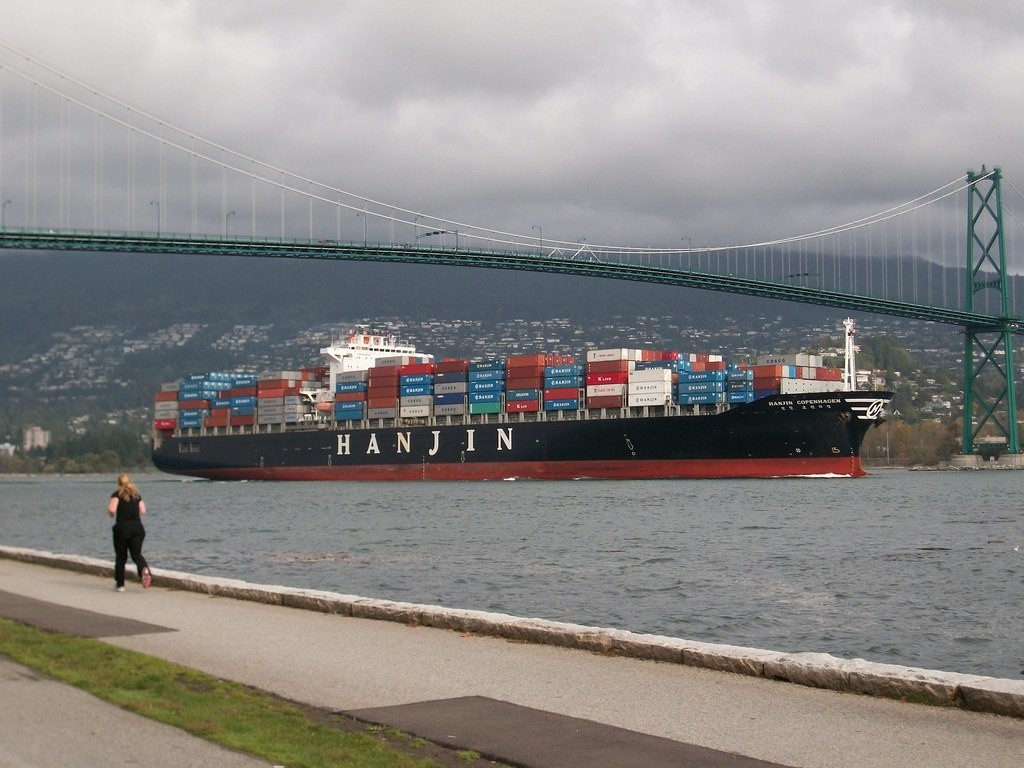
108,473,152,593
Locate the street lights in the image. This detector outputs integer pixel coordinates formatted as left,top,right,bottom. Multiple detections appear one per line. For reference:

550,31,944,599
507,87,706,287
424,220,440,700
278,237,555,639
681,237,692,272
150,200,160,238
532,226,543,254
357,212,366,246
226,211,236,238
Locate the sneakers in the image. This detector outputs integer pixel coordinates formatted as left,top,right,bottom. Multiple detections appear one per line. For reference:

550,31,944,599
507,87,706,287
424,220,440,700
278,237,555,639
117,587,125,593
142,567,151,589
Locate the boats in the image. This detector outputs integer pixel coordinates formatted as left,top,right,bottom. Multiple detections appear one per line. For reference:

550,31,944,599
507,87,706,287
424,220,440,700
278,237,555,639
150,319,896,479
